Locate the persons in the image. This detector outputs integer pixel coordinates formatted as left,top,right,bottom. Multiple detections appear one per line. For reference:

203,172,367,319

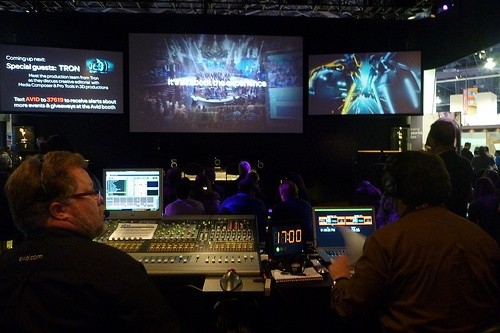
163,159,399,227
425,119,474,218
0,150,182,333
327,150,500,333
0,120,101,189
460,141,500,243
139,60,301,132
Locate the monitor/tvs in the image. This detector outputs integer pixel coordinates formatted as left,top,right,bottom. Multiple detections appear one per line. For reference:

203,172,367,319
101,168,165,218
312,205,377,250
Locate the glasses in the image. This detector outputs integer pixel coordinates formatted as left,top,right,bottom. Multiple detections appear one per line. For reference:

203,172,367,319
63,189,101,200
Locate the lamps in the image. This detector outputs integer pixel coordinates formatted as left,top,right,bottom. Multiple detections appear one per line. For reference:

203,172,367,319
443,50,497,74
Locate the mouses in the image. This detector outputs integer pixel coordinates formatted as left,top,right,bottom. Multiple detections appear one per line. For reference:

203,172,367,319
219,270,241,292
